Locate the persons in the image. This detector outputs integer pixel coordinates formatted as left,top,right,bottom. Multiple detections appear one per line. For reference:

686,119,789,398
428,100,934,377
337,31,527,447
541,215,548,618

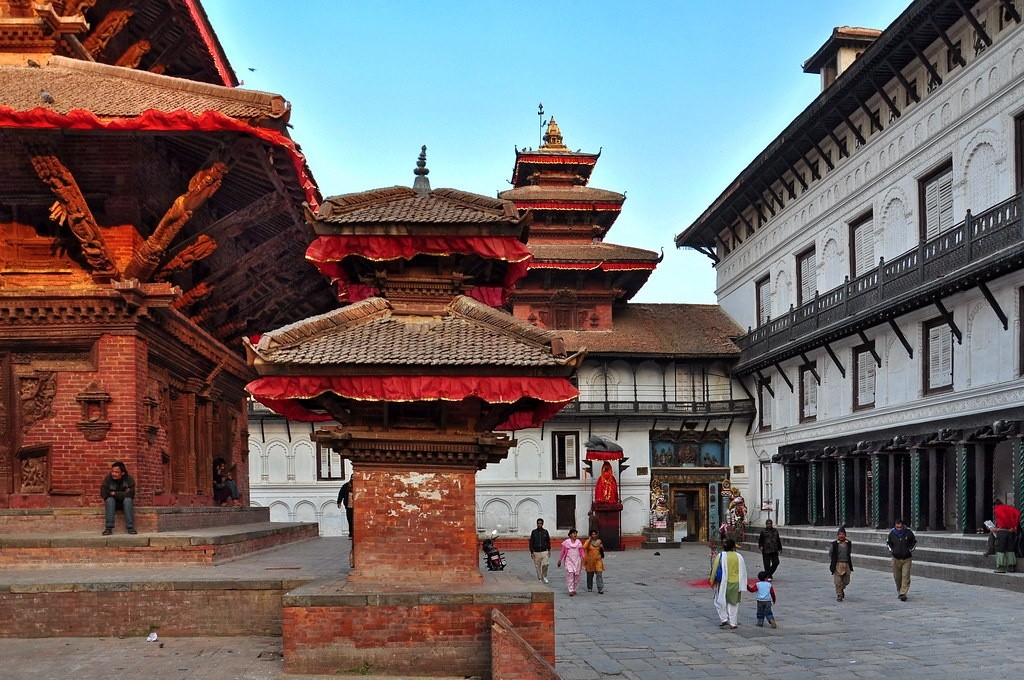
530,518,551,583
829,528,854,602
337,473,353,540
583,530,605,594
558,529,585,596
747,571,778,628
886,519,917,600
985,499,1020,573
213,458,241,507
100,462,137,535
758,519,782,581
710,539,747,629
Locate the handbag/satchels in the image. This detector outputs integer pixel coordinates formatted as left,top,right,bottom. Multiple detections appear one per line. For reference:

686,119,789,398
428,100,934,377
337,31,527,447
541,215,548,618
715,553,722,583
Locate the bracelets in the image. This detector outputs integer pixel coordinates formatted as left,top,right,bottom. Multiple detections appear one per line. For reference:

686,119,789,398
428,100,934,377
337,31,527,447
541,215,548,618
113,492,114,495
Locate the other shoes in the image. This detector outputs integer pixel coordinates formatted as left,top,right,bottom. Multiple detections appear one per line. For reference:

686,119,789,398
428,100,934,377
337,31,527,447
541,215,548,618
730,625,737,629
1006,566,1017,573
719,621,728,628
233,502,241,506
756,623,764,627
994,567,1006,573
587,589,592,592
598,588,603,594
898,596,903,599
569,592,574,597
574,592,578,595
837,596,844,602
772,621,777,629
901,595,907,601
221,502,230,506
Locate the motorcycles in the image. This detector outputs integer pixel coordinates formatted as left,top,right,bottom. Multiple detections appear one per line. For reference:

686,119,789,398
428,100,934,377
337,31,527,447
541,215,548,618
480,530,507,572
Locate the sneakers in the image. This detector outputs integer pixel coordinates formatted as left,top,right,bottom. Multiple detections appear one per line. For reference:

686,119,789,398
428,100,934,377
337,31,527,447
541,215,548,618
543,577,548,584
127,527,137,534
102,528,112,536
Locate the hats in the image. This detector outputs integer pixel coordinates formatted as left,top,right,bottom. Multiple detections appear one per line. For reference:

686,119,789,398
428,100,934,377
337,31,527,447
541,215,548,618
838,527,846,533
